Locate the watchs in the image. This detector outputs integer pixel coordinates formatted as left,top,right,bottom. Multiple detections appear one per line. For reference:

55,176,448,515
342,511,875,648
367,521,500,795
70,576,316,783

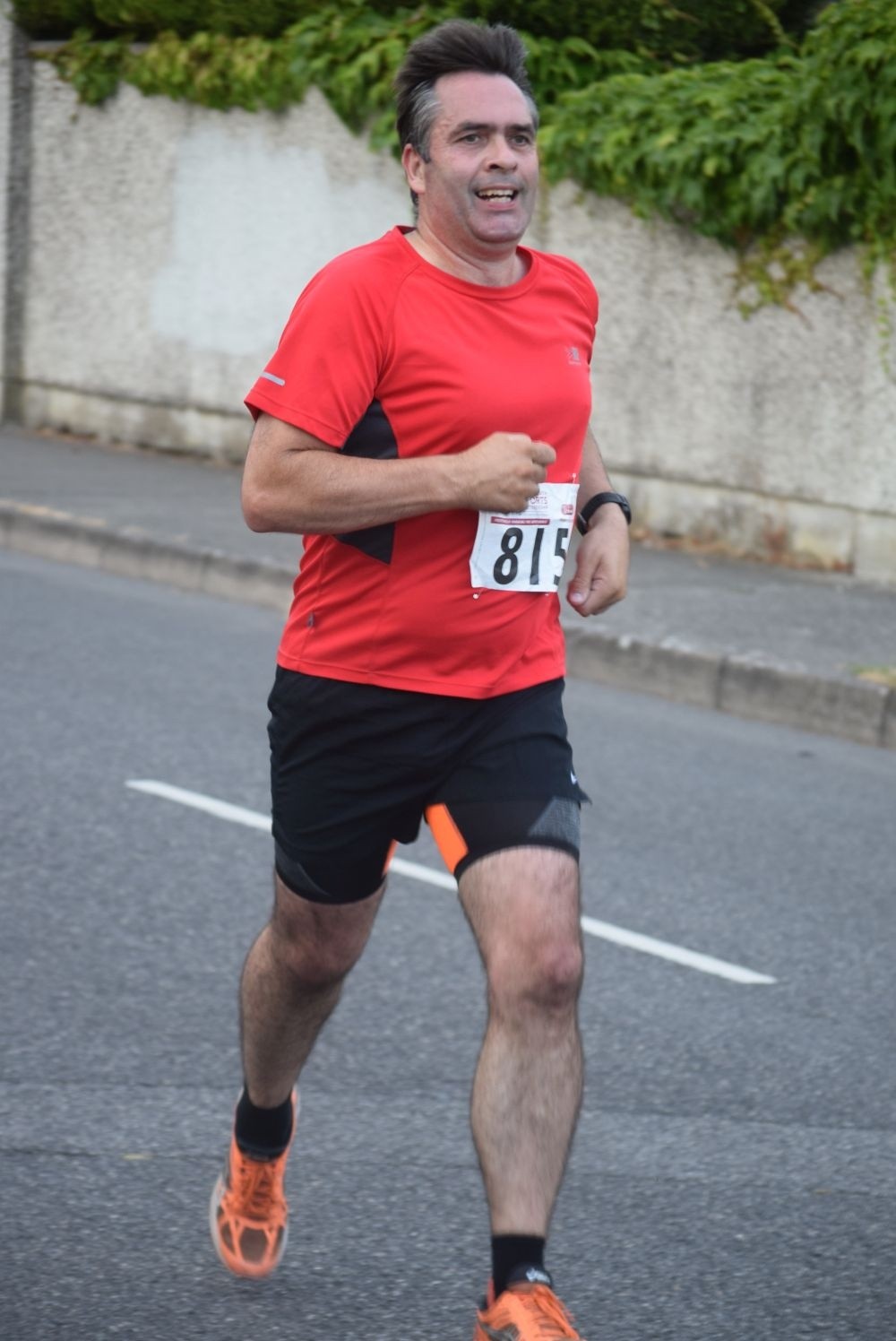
575,492,632,535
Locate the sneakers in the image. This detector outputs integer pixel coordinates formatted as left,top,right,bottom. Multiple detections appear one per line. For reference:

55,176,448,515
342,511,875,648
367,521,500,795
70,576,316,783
474,1281,583,1341
209,1088,299,1279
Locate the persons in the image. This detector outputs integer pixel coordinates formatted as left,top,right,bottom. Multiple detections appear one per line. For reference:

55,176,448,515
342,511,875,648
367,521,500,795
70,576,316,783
201,15,639,1341
